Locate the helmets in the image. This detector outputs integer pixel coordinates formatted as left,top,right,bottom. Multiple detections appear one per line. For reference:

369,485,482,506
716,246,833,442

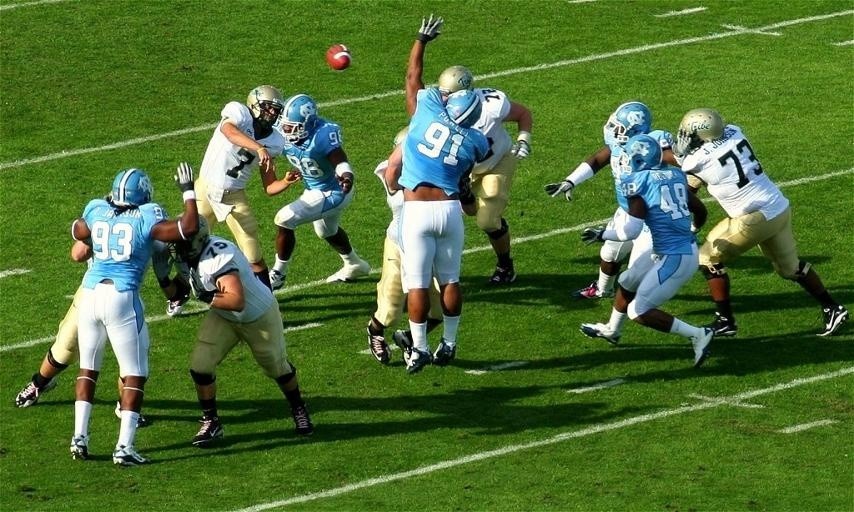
603,100,663,178
679,109,725,145
246,84,319,145
107,169,153,207
438,64,483,130
166,213,210,264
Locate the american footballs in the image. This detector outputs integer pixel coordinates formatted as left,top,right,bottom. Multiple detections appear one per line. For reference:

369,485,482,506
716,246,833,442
326,45,350,70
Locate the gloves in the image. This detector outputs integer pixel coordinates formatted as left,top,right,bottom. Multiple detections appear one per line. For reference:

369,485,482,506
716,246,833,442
188,268,207,300
543,179,575,202
416,13,445,42
174,162,195,192
510,140,530,160
581,227,604,246
676,127,692,155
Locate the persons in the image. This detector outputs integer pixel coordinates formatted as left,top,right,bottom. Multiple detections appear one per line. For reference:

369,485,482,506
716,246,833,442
269,93,370,290
69,162,199,466
674,110,848,338
166,85,302,319
16,239,150,428
153,211,313,447
580,135,715,368
543,102,682,299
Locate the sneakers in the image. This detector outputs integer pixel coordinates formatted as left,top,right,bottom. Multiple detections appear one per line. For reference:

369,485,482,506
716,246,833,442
269,267,287,292
292,402,313,436
700,312,736,337
112,443,151,466
17,378,57,409
579,322,620,346
487,260,517,284
166,295,190,317
570,281,616,301
192,415,224,447
691,326,715,368
816,304,849,338
70,434,90,459
115,400,146,428
367,320,457,374
326,260,371,283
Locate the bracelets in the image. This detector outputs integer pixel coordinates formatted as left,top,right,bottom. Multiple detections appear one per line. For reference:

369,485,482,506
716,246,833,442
182,190,196,204
334,162,353,177
256,148,268,153
